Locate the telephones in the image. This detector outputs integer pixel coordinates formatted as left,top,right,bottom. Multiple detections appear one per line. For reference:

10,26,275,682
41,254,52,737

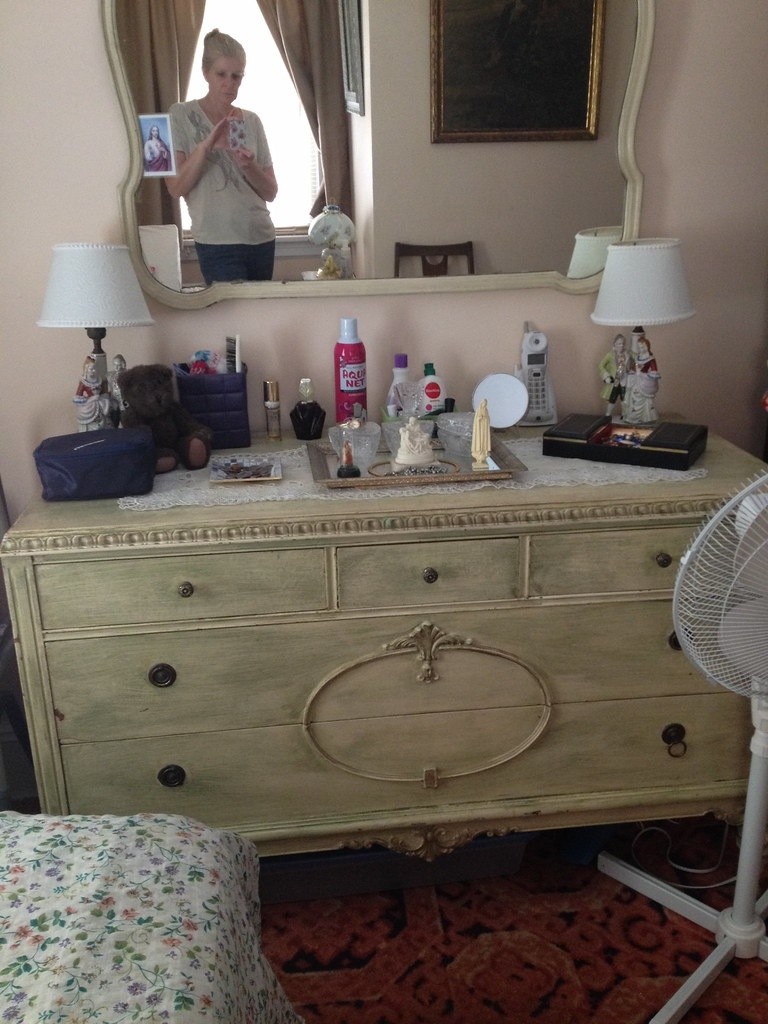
514,321,558,426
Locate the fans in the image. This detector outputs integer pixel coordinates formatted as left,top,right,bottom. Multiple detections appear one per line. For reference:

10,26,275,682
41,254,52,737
596,470,768,1024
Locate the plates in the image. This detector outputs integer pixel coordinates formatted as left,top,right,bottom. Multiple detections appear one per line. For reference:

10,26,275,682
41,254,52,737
469,373,529,429
210,457,283,483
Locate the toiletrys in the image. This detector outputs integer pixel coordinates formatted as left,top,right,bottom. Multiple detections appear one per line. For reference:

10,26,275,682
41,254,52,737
386,353,448,417
333,316,369,424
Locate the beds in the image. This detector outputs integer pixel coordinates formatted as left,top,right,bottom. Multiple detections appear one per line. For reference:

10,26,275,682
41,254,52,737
0,811,298,1024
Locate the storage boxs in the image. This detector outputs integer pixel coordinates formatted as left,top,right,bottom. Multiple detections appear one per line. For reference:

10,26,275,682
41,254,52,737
175,362,250,451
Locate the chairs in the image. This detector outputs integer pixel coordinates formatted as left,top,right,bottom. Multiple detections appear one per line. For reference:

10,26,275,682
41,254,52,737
391,243,475,277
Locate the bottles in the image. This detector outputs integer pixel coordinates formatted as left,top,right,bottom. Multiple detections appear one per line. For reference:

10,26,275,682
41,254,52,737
416,362,447,416
385,352,414,411
334,317,368,423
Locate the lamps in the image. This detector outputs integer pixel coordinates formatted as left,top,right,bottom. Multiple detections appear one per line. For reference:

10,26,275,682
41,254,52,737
306,204,356,275
566,226,622,280
34,240,152,429
590,239,695,421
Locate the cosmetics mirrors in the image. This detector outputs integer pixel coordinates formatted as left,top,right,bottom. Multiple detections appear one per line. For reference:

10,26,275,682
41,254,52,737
471,372,529,429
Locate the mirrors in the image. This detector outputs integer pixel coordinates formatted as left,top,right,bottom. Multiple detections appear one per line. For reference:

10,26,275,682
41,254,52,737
102,1,654,297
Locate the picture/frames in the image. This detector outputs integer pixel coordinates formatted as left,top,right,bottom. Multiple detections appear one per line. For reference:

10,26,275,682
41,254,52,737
335,2,366,118
428,1,605,147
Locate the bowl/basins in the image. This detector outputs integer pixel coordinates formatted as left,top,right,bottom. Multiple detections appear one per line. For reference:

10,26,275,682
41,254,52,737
382,420,434,456
328,421,380,464
437,412,481,456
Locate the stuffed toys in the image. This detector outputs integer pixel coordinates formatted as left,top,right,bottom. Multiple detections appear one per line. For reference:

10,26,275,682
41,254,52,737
118,363,213,474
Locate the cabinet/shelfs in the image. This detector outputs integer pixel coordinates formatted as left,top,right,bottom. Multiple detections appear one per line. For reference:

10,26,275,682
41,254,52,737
0,427,768,857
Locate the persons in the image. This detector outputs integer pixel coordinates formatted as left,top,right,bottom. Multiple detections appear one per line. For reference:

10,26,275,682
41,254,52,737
163,27,278,283
472,398,491,463
143,125,171,171
74,356,115,433
599,334,633,418
399,416,430,442
621,338,660,425
104,354,127,425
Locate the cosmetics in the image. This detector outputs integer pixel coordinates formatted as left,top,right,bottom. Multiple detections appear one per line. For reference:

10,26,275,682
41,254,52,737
264,380,281,441
384,353,446,418
290,378,325,441
334,317,367,423
